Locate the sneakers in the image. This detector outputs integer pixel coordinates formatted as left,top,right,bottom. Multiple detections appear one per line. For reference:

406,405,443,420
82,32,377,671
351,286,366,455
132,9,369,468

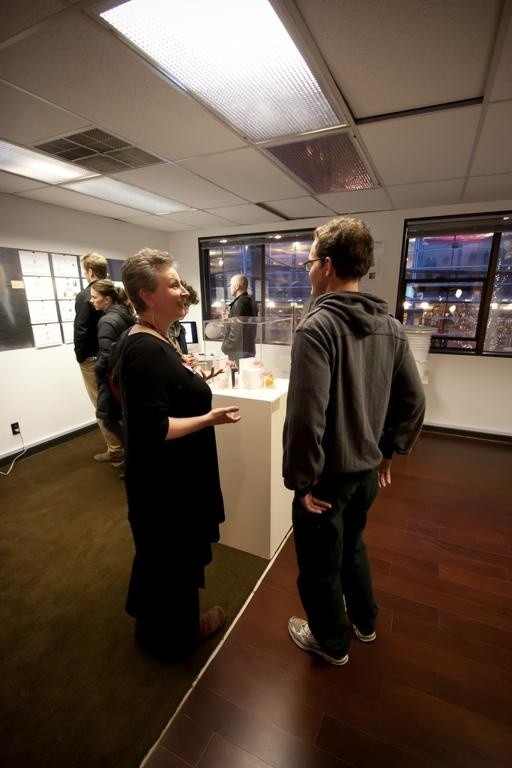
342,595,376,642
288,615,349,666
94,454,123,462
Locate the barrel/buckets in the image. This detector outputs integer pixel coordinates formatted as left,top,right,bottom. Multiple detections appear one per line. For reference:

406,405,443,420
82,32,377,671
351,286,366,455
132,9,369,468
407,333,432,382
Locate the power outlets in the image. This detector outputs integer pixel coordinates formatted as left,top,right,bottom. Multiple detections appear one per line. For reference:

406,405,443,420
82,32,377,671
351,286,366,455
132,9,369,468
11,422,22,435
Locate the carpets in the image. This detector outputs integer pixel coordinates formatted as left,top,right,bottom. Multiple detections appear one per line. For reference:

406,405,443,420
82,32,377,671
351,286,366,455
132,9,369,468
1,424,296,767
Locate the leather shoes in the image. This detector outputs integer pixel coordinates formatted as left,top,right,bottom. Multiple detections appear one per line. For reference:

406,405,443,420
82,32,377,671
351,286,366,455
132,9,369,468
198,606,225,640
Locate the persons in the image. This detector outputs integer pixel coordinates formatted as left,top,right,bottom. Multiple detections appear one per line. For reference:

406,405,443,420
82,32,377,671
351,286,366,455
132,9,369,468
281,216,427,667
75,253,123,464
171,280,199,366
221,275,258,387
111,246,225,661
89,278,137,481
0,261,35,354
